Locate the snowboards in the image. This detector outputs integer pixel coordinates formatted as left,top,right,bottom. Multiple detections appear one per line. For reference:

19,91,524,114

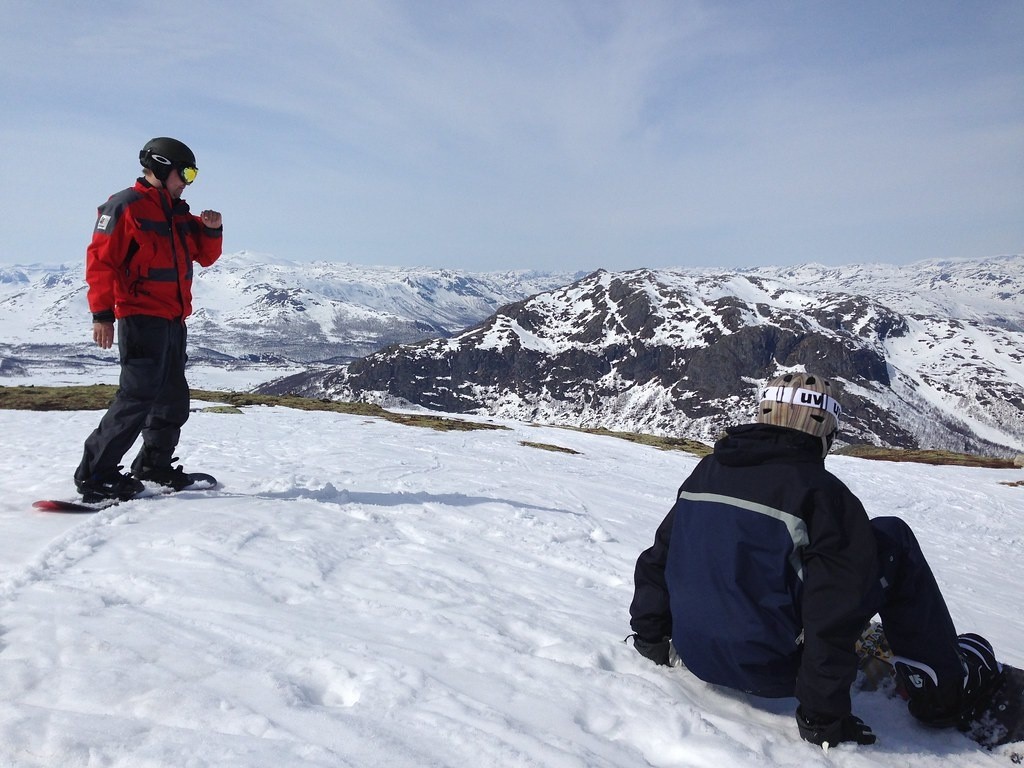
32,471,219,514
855,621,1023,768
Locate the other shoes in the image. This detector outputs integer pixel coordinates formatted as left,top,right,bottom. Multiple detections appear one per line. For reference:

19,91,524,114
889,632,1001,726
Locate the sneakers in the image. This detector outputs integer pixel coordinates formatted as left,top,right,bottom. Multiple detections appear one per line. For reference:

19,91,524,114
131,452,196,489
73,471,145,498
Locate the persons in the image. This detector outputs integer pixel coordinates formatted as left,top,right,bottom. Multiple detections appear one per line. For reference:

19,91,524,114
628,369,997,748
70,136,225,503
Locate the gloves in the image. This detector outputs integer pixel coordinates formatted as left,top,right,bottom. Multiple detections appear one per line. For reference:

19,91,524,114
632,634,683,668
795,704,875,749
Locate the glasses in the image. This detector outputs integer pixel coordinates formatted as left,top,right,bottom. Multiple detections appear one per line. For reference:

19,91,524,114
178,164,198,185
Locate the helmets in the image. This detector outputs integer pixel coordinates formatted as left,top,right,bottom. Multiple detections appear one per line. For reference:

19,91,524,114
139,137,196,180
761,372,840,436
